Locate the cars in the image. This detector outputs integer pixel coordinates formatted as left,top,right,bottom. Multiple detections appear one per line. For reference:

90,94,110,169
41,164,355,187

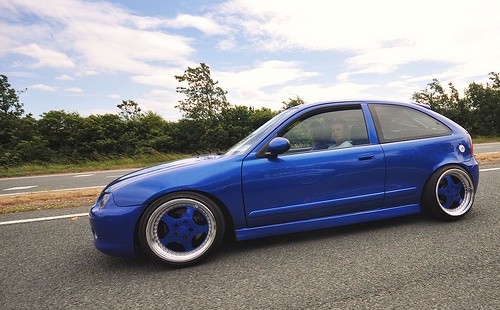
88,99,481,270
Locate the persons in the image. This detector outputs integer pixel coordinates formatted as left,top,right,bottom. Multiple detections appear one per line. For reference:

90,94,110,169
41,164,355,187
328,119,353,148
309,121,329,150
